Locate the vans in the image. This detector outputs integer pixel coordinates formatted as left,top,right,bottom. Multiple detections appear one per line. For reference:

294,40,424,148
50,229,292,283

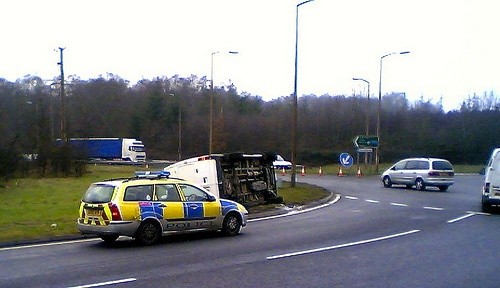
164,150,283,208
479,148,500,212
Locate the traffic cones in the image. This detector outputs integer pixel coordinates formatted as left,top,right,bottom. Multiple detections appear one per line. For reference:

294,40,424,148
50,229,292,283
280,166,286,176
355,166,362,178
300,166,306,176
317,165,324,176
337,166,344,177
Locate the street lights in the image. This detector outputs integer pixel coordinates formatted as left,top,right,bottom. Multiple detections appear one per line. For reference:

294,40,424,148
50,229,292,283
290,0,315,187
208,51,238,159
352,77,370,165
375,51,410,172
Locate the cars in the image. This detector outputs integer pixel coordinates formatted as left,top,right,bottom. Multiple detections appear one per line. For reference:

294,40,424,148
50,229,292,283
75,170,248,247
272,155,292,171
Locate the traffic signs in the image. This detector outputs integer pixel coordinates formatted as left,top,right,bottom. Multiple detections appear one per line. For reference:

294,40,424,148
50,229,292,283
353,135,379,148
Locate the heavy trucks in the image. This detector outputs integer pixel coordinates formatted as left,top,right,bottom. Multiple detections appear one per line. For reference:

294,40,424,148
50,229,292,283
56,137,147,165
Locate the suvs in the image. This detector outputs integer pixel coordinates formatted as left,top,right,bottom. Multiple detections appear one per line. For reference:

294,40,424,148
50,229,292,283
380,157,455,192
